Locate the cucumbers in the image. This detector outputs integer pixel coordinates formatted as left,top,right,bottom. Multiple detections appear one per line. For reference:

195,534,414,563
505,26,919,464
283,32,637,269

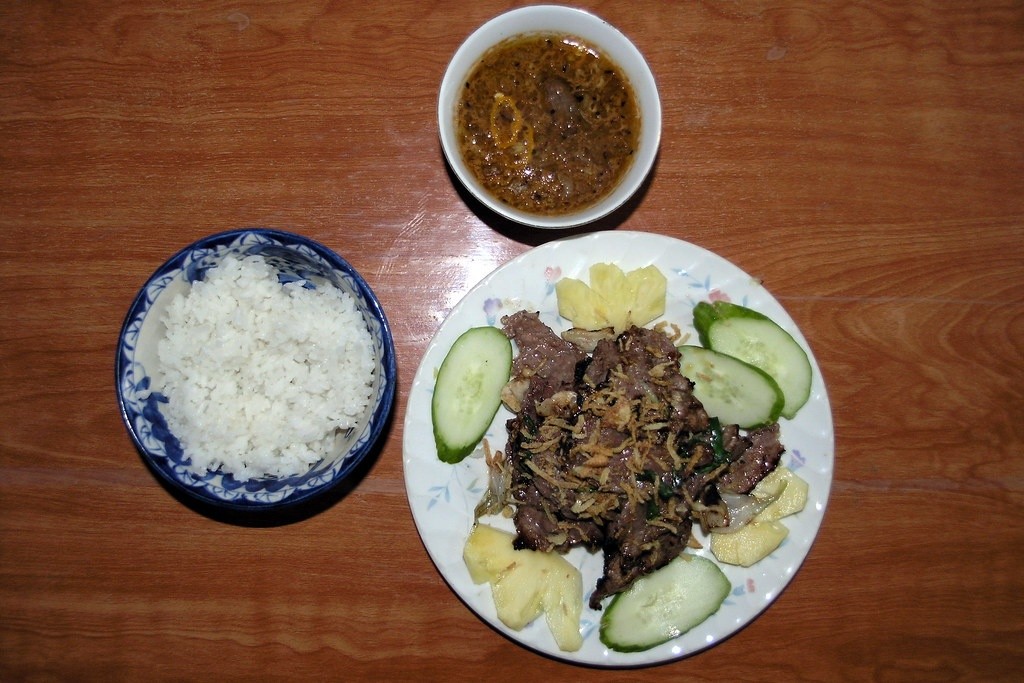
431,327,513,465
591,545,732,651
694,295,812,423
673,340,784,432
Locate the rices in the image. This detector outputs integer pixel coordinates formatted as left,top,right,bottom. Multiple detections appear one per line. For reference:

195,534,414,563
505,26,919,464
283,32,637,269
156,250,383,483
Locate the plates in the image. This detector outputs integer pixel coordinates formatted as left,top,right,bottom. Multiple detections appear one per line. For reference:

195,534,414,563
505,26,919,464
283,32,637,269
403,230,836,667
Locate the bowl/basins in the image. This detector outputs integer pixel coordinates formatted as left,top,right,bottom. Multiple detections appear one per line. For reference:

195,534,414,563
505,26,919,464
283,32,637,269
439,4,662,229
117,228,396,521
